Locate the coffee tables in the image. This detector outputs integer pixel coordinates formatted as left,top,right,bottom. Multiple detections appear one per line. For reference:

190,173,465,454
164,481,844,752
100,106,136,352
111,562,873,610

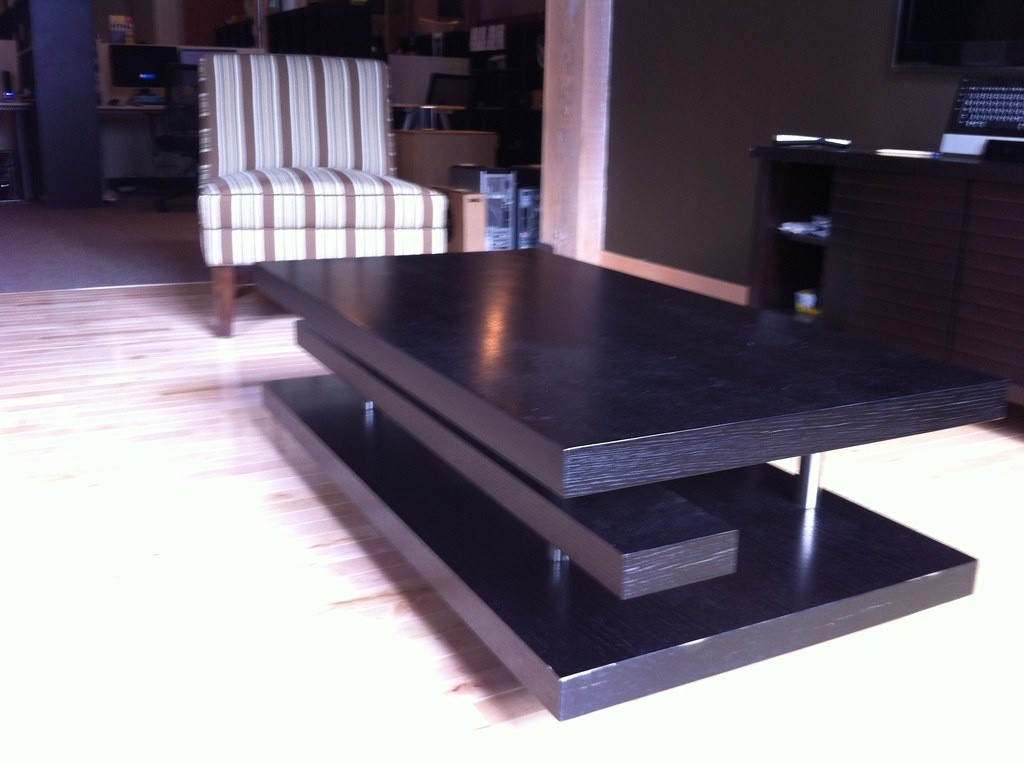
252,248,1011,722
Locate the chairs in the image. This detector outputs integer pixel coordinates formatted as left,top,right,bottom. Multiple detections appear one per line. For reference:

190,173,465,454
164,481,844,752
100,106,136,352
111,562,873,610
199,54,450,338
131,64,199,214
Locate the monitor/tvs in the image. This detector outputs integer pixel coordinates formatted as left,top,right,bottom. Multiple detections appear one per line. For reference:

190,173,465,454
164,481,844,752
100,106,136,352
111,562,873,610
938,71,1024,156
107,44,179,104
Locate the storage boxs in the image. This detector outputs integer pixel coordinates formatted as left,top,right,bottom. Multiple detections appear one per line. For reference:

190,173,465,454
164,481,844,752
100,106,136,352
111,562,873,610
428,163,541,253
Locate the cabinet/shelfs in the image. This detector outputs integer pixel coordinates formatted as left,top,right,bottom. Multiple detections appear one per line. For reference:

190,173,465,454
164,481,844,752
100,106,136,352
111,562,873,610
746,143,1023,407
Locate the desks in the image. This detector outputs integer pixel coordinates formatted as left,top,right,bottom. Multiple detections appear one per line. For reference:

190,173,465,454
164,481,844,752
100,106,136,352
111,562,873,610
0,102,37,202
96,104,170,205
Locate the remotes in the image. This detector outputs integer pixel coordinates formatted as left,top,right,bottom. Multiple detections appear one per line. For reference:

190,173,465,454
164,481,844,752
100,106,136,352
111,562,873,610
770,134,853,148
